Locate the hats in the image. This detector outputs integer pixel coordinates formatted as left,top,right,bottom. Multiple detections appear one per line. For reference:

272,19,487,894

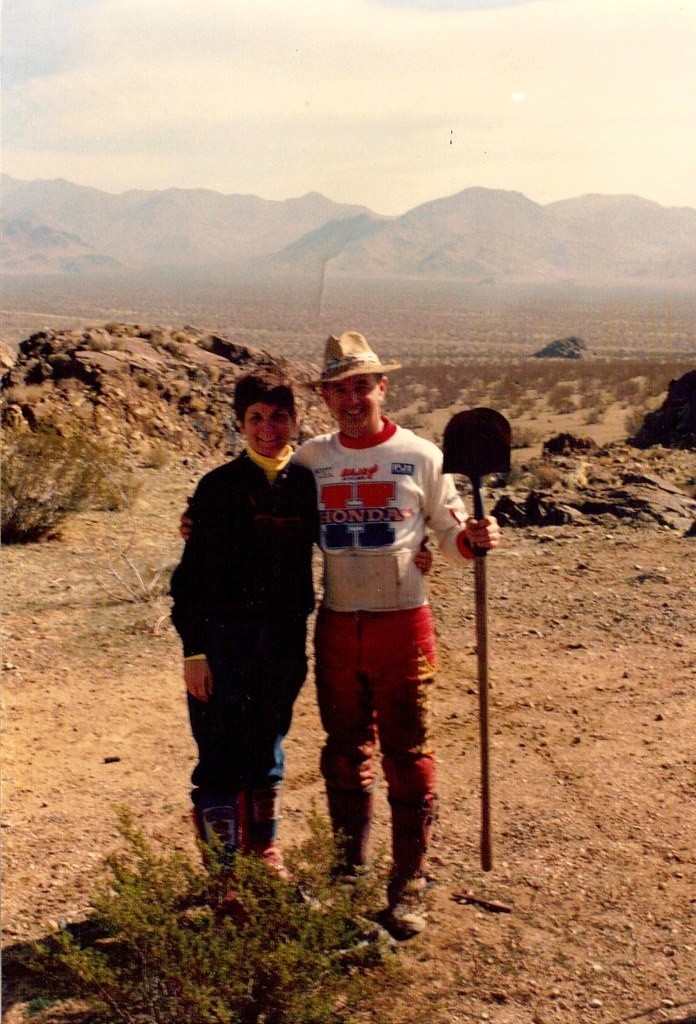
305,331,402,388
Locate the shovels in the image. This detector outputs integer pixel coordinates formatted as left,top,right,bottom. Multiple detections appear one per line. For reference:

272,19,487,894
440,406,513,873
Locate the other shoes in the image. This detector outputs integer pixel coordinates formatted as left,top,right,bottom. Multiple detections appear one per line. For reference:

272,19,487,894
335,866,378,895
258,847,292,888
387,876,428,932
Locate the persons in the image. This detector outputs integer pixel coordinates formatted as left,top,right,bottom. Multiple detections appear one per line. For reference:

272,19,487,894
177,332,504,932
165,369,434,904
